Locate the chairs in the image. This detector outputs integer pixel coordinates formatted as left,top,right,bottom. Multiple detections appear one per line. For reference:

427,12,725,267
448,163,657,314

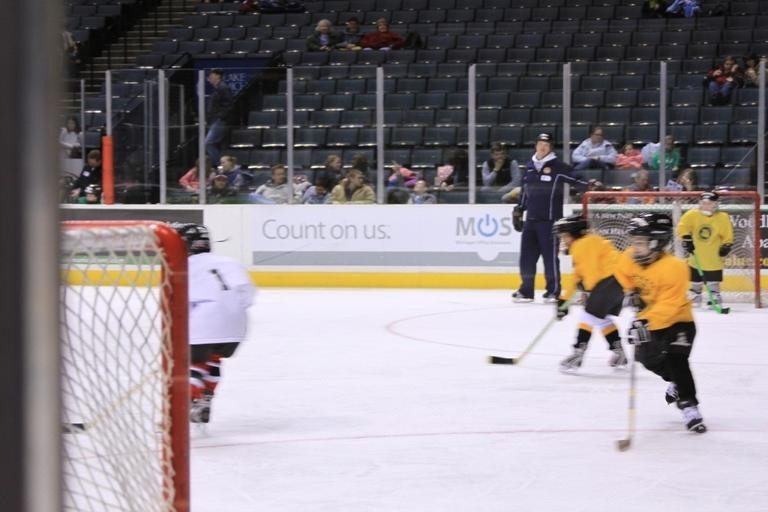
55,0,767,203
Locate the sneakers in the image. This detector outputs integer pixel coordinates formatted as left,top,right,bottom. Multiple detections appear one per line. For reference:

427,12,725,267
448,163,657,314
561,352,582,367
665,380,704,430
610,356,628,367
543,291,560,298
512,290,533,299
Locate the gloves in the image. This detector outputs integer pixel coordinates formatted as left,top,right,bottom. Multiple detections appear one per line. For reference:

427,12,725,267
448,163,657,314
719,243,731,256
682,235,694,251
628,319,650,344
558,301,568,319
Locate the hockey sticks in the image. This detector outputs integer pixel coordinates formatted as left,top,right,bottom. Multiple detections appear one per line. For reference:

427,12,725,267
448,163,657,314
486,316,556,365
614,351,638,450
691,251,730,314
60,367,161,433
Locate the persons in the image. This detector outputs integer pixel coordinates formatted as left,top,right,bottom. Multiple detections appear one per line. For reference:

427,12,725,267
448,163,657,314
511,132,602,299
706,56,743,105
613,212,703,431
176,224,255,425
549,213,629,370
573,127,701,203
388,143,520,203
177,153,376,203
361,18,405,50
676,192,732,306
60,118,103,203
334,17,366,50
743,52,768,86
307,19,336,52
209,68,232,123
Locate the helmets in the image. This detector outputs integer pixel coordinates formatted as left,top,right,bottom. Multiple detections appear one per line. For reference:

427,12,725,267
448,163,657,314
552,216,588,238
627,213,672,265
177,223,210,255
700,192,719,201
536,133,552,141
